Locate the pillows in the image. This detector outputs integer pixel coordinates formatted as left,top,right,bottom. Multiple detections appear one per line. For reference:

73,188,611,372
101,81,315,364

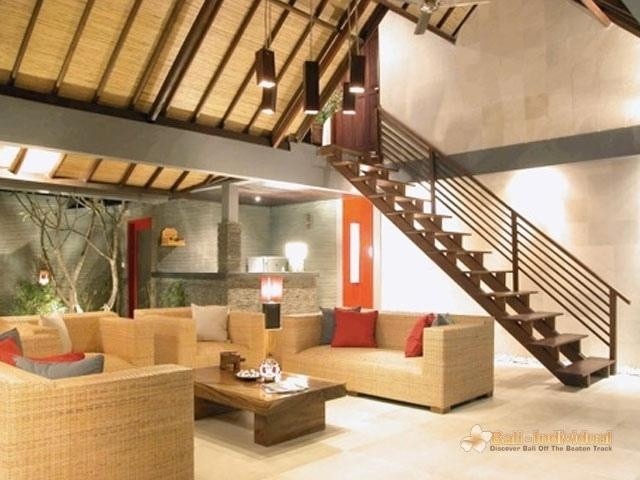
332,307,379,348
0,327,22,350
13,355,104,379
0,336,23,365
1,315,72,358
191,304,232,343
405,313,435,357
319,306,361,346
431,312,454,328
28,353,84,363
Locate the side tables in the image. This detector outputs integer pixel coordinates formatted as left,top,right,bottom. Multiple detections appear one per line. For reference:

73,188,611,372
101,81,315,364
266,328,281,358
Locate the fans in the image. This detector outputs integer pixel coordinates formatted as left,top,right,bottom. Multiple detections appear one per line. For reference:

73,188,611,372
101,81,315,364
406,0,491,34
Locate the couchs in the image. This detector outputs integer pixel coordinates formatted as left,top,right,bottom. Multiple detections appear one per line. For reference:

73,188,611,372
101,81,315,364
280,312,495,414
0,362,195,480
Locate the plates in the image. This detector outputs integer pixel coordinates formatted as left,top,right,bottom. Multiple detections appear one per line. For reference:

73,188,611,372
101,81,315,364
234,369,260,379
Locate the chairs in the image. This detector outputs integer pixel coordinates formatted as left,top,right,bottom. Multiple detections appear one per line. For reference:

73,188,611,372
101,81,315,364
134,307,266,421
1,311,153,377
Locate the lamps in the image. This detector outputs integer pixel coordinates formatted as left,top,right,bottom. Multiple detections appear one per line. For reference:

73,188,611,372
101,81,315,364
261,274,283,329
254,0,365,116
